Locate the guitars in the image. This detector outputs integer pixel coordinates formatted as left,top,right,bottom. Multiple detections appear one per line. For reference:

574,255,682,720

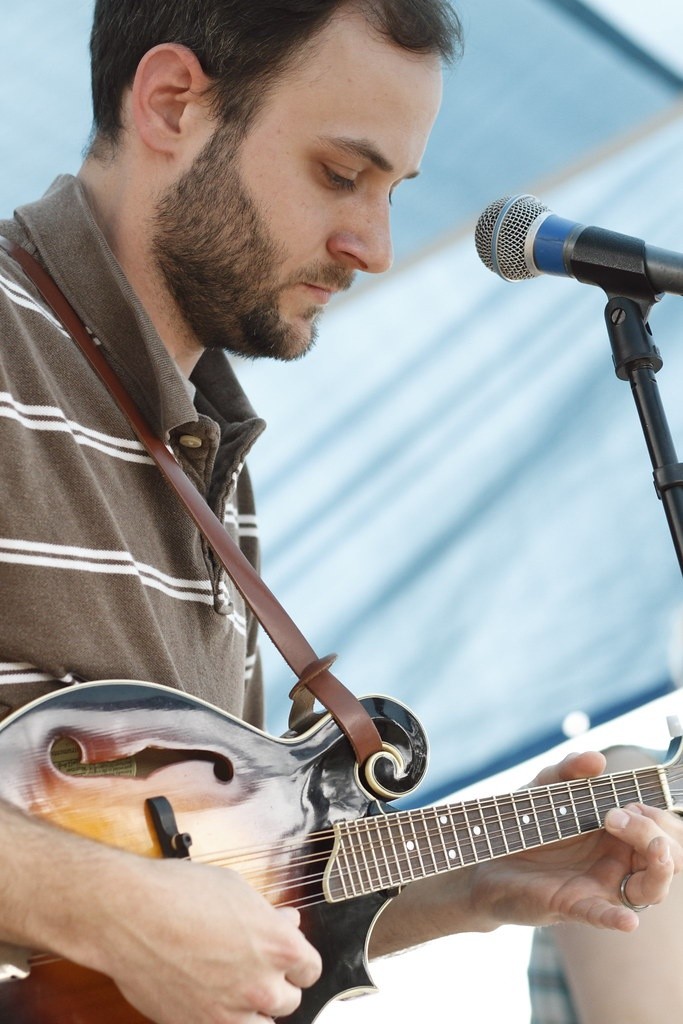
0,676,683,1024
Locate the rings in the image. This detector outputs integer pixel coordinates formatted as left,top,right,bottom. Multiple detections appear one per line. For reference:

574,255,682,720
621,872,652,912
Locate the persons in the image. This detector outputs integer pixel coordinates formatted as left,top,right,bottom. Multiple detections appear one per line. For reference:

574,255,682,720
0,0,683,1024
526,746,683,1024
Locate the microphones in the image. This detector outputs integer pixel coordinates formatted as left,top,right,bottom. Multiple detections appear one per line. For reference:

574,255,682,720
474,191,682,296
